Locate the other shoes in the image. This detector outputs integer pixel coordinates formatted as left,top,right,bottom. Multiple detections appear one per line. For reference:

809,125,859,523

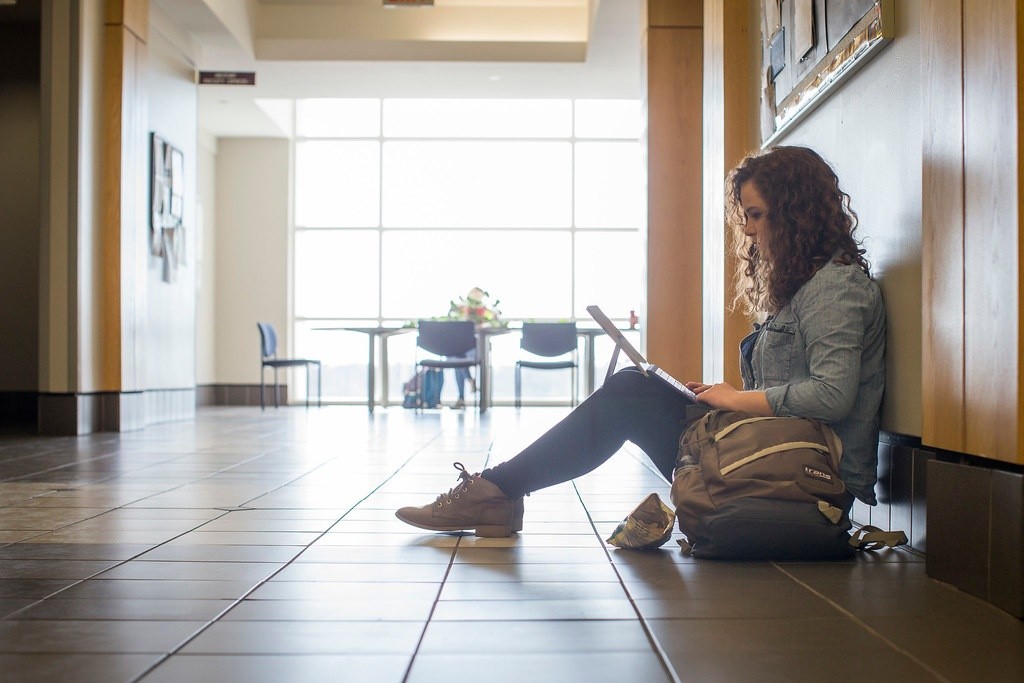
470,378,476,392
449,399,466,409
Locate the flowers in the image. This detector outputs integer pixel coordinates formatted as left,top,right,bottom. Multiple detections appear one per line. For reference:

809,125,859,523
403,287,508,328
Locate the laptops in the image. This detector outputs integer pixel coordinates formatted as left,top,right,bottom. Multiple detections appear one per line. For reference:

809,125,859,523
585,305,699,403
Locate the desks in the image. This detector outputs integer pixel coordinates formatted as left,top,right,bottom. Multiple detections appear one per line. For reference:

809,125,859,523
310,327,605,414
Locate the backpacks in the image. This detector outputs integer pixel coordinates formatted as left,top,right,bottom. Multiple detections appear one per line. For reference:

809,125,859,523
670,407,857,563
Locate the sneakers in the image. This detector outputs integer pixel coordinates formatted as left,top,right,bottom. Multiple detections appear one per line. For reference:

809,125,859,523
395,462,524,538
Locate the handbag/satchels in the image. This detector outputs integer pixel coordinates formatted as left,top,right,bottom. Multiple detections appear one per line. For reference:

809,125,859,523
401,363,444,408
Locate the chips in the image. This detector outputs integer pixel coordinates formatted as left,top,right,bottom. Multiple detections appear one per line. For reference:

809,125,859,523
637,520,665,536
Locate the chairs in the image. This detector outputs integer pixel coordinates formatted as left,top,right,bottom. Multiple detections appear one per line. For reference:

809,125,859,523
255,321,321,410
414,320,482,414
515,322,579,408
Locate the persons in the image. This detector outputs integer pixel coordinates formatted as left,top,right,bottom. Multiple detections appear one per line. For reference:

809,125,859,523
395,146,887,545
404,290,491,411
